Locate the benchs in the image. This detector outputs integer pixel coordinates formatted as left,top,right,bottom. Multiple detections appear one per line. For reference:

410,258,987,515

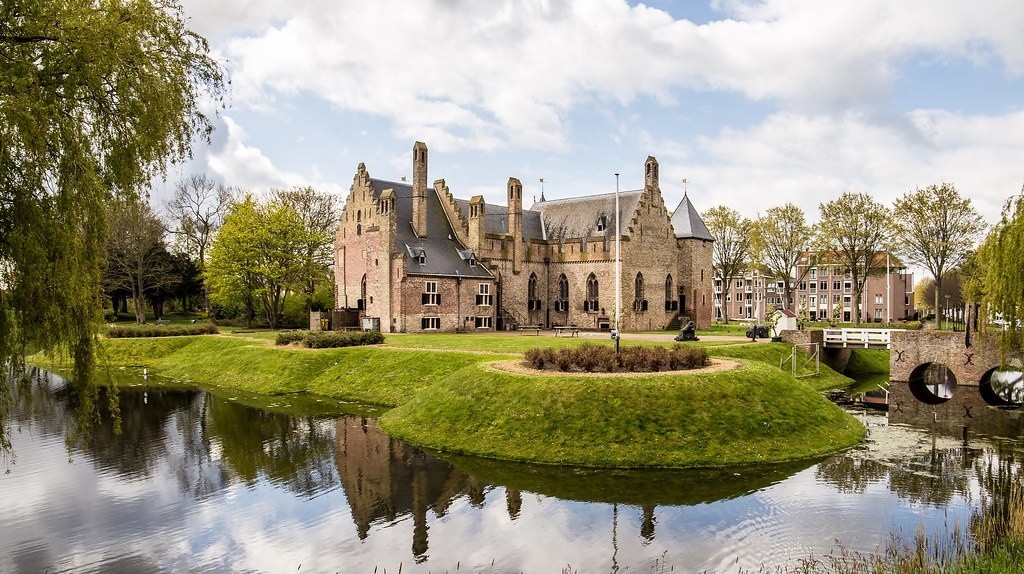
551,327,584,338
518,326,542,336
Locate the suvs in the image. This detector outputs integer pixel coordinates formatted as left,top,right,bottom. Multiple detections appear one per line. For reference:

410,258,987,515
746,326,769,338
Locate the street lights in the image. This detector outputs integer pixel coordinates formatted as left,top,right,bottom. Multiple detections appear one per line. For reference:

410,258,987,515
945,294,950,323
884,248,890,326
917,302,921,322
614,173,621,366
